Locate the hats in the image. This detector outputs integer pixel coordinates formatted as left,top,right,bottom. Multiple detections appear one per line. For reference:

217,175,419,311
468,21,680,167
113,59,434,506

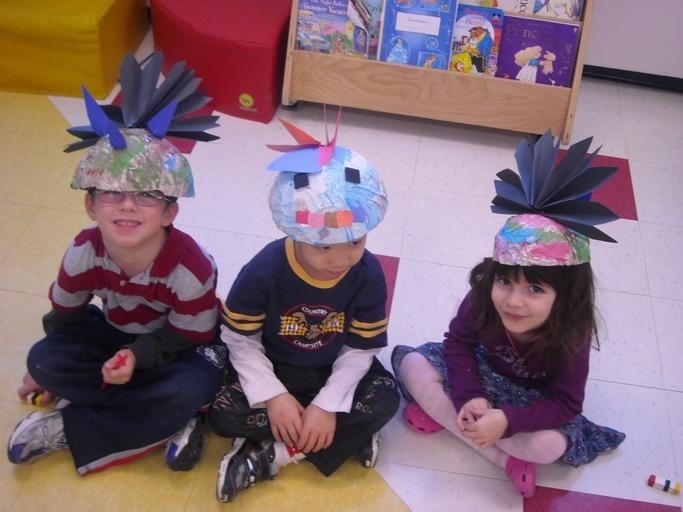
492,210,591,268
70,126,195,197
268,144,388,246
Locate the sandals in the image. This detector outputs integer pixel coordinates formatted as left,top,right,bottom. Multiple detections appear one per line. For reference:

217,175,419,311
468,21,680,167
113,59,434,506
506,453,534,499
403,398,446,432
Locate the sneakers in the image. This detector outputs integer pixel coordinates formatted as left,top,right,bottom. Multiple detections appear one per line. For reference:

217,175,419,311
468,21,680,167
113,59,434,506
164,411,206,472
215,435,275,504
357,430,379,470
8,397,73,466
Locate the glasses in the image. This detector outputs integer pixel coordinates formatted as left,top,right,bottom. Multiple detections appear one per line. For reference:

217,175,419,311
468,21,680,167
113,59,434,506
88,186,173,208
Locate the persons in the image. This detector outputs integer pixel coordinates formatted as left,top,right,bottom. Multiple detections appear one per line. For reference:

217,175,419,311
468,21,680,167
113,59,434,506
7,51,229,477
390,129,626,501
205,102,401,506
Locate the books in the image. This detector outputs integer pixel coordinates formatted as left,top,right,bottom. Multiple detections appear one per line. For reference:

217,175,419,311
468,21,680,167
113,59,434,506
381,0,457,71
491,11,583,87
445,4,503,83
292,0,382,63
457,0,589,27
